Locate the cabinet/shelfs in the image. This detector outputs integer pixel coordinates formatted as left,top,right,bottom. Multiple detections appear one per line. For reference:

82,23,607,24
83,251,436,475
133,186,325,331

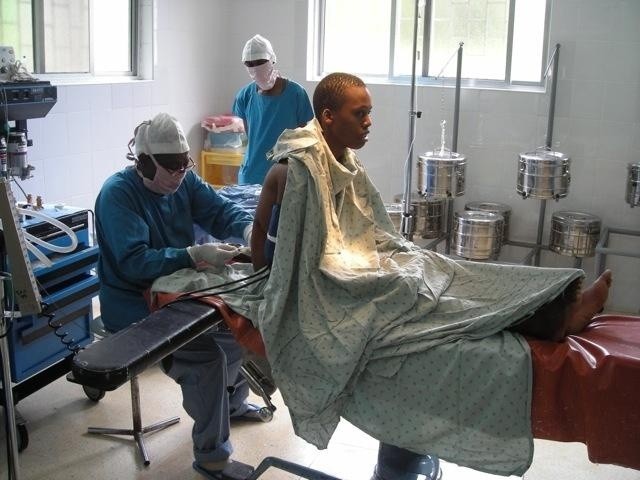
199,149,246,193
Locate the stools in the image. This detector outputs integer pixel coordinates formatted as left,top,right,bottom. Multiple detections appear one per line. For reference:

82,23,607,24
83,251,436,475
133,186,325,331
80,314,183,466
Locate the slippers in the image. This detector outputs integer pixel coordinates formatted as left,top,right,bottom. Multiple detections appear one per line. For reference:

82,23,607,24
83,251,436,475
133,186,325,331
230,403,265,423
191,459,256,480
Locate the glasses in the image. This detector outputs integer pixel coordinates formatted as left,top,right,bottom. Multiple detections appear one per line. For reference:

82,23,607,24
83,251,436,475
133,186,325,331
153,156,197,177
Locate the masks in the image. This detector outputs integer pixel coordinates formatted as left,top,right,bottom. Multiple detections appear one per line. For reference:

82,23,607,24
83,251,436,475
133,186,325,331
243,60,282,92
141,165,188,195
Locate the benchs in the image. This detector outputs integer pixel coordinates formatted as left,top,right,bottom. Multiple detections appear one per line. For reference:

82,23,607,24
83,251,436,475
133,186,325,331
243,308,639,471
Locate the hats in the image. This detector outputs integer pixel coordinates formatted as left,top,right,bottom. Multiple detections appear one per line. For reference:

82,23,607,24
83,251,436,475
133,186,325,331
133,112,191,156
241,34,277,64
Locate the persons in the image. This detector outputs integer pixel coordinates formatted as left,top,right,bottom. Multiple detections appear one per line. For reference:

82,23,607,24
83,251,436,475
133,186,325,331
234,32,314,187
91,113,275,479
249,70,615,343
